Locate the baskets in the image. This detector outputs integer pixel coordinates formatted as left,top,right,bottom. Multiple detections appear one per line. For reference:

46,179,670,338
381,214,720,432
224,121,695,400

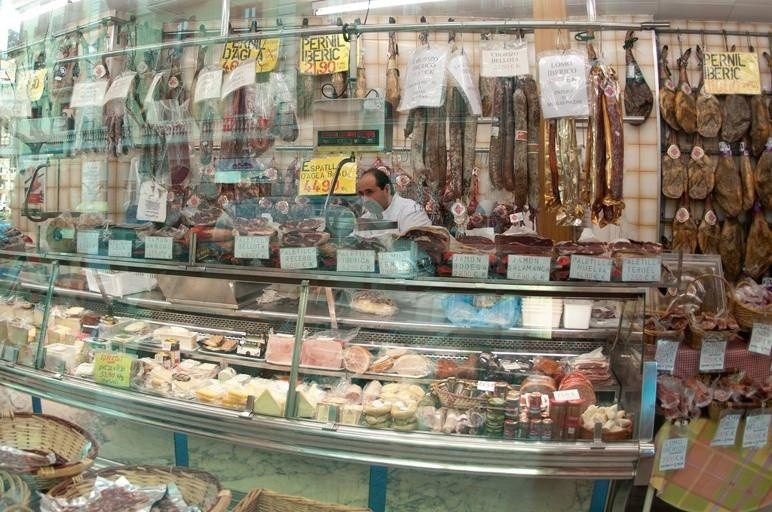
430,375,491,412
226,487,372,511
1,383,99,491
683,273,738,350
628,264,688,347
38,463,220,511
727,278,771,328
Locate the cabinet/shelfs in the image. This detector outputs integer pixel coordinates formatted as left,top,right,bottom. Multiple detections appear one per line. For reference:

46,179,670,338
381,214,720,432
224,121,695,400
0,252,656,512
657,26,772,254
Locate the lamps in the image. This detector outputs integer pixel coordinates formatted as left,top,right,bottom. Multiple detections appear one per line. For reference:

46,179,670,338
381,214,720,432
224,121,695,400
313,0,365,16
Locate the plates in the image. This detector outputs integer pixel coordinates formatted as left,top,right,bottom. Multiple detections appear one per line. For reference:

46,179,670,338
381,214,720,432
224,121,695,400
346,364,429,379
198,348,265,363
136,332,199,352
266,359,345,372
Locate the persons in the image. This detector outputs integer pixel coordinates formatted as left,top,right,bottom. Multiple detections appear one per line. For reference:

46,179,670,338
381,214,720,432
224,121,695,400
348,168,433,239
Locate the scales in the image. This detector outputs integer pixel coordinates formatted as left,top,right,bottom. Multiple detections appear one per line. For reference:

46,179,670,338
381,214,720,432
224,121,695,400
15,117,80,222
311,99,399,231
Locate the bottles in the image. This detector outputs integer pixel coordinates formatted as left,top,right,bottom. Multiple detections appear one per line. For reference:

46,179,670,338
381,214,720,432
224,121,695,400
165,338,180,367
542,418,553,441
504,419,516,438
160,352,170,368
495,383,507,399
529,418,542,442
530,391,541,410
551,398,567,440
564,399,582,440
